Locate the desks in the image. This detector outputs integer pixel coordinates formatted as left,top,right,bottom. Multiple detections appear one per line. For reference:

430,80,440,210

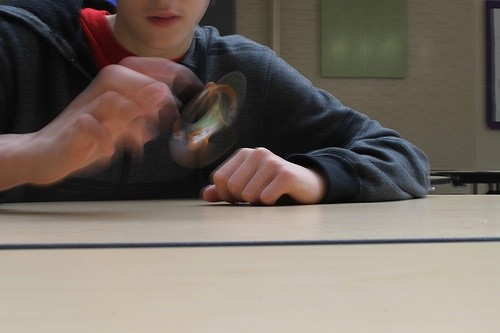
2,192,500,332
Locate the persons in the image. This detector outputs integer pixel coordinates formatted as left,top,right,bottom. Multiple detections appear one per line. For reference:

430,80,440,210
1,0,431,213
